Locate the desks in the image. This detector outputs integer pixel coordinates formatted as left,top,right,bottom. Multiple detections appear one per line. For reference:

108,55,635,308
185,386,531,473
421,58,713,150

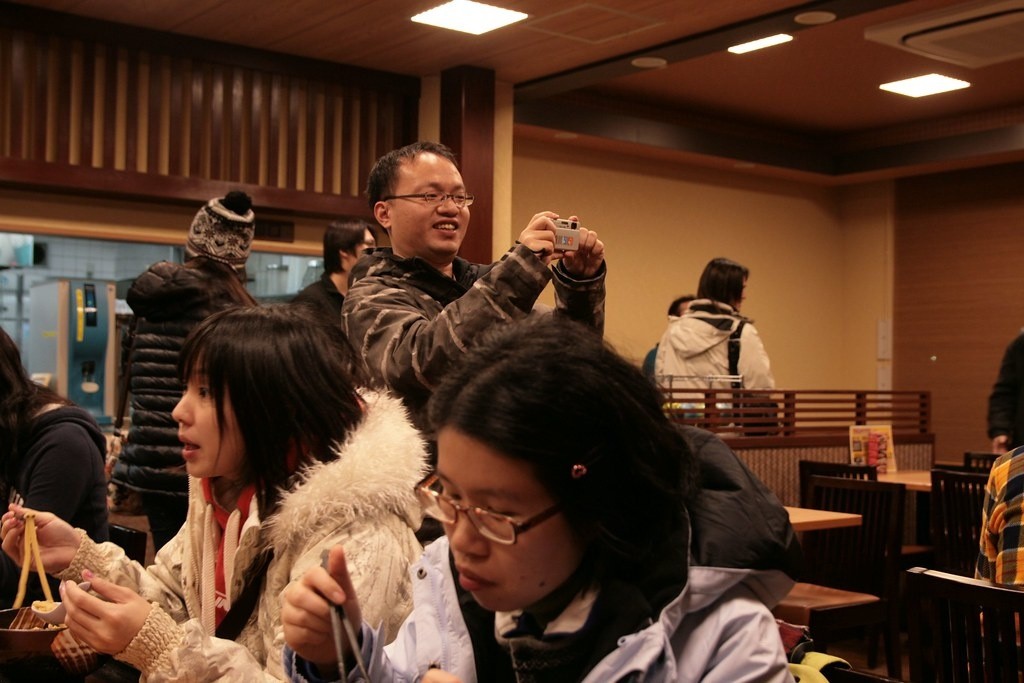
782,505,862,546
851,470,986,545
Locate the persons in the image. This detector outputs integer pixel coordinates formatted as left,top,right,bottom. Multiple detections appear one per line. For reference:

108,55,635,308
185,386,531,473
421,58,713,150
341,141,607,545
111,190,268,682
294,219,376,305
1,323,105,681
278,313,790,683
1,305,424,683
641,258,775,436
965,333,1024,682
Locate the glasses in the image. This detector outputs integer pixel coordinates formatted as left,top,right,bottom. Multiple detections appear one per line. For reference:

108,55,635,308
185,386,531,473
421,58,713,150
383,191,475,207
414,469,566,545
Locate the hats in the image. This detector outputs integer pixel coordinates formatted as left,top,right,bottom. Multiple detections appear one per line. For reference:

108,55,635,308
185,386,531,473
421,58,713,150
186,191,256,268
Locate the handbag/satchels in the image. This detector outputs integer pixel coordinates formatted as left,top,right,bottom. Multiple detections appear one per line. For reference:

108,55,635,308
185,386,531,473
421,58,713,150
104,430,148,517
728,317,780,437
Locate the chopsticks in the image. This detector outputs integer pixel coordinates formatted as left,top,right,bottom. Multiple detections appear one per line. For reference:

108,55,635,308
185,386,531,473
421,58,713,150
322,551,375,683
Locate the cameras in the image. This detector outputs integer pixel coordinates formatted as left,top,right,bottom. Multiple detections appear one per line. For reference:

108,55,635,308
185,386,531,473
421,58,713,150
548,217,580,250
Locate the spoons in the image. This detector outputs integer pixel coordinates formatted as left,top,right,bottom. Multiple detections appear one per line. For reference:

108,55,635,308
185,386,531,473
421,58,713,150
33,582,90,625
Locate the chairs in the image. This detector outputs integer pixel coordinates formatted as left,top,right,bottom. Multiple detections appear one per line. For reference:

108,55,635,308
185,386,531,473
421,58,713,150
786,451,1024,683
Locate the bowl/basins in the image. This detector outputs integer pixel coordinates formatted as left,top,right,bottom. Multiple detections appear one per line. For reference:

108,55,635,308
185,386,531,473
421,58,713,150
0,607,68,664
